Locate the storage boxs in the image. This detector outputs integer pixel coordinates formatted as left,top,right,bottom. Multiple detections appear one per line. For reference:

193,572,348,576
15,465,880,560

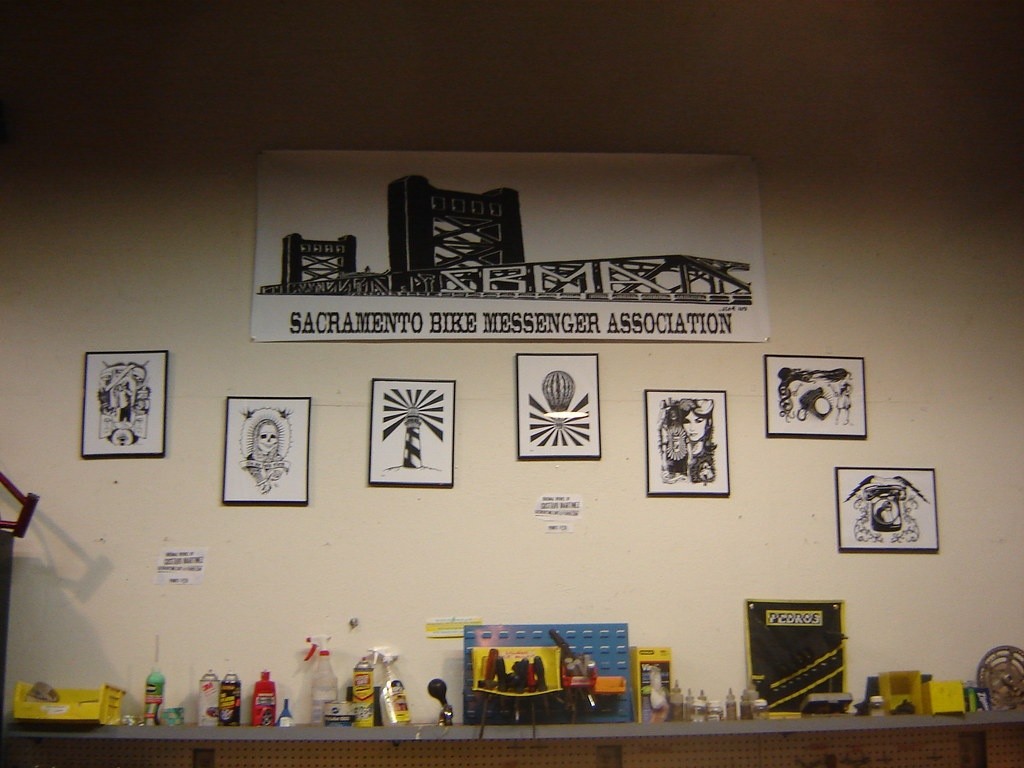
630,645,673,723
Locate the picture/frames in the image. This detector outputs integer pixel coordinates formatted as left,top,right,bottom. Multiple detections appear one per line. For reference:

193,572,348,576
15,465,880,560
645,389,730,497
515,353,601,459
223,397,311,506
369,378,456,487
834,467,939,551
763,354,867,439
82,351,166,458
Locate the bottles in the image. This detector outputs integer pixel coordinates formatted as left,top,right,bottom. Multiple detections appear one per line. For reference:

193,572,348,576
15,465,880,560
670,679,770,724
252,670,276,728
220,672,241,726
196,669,221,726
143,665,166,725
868,696,890,717
352,657,375,727
